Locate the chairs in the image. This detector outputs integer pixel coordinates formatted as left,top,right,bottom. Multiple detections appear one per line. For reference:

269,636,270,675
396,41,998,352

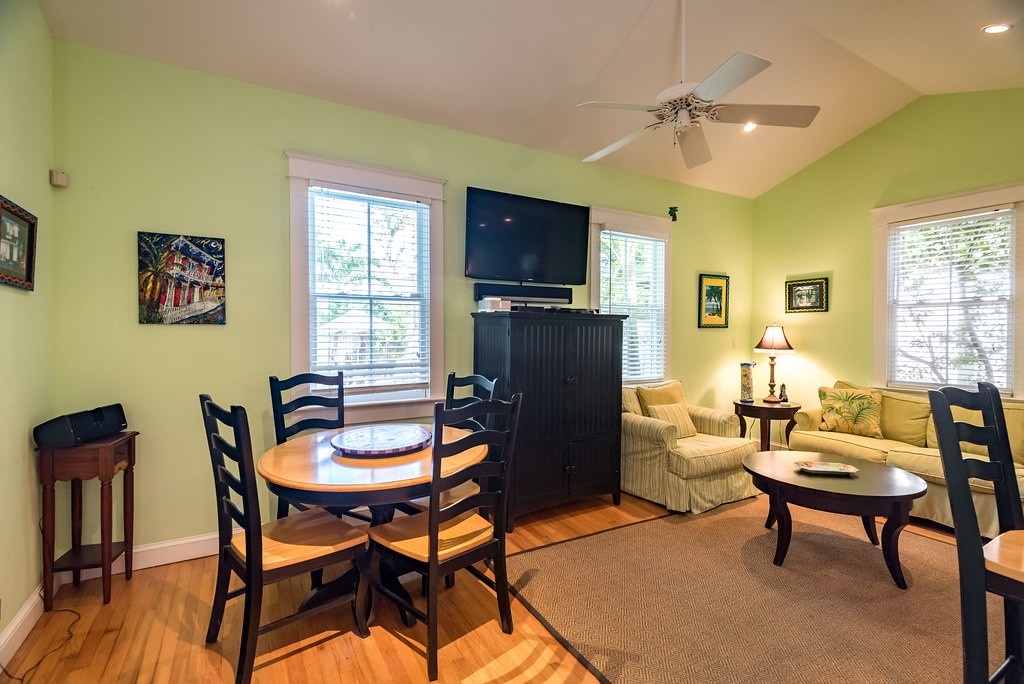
269,371,384,590
353,392,525,682
927,381,1024,684
199,394,381,684
378,371,502,589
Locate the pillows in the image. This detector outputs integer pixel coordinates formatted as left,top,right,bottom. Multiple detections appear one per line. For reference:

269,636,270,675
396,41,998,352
646,403,697,438
636,381,683,417
818,385,885,439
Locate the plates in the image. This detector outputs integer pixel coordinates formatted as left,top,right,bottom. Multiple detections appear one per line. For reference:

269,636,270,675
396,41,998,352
793,462,859,476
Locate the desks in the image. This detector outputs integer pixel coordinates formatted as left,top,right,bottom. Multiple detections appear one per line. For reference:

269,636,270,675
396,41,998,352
733,398,801,450
256,424,487,629
40,432,140,611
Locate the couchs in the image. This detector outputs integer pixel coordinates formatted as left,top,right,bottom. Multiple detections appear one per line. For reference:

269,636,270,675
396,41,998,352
621,379,760,515
788,378,1024,537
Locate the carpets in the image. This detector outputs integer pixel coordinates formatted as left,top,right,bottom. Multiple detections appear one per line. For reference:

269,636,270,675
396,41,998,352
484,511,1007,684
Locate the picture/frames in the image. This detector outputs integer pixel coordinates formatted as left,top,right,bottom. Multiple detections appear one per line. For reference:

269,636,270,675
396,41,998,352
698,274,731,327
785,277,829,313
0,194,38,291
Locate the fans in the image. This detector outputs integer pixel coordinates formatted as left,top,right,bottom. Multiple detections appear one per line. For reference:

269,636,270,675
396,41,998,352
576,2,822,168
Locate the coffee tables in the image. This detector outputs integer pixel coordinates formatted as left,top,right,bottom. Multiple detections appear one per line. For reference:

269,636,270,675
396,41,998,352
743,452,927,589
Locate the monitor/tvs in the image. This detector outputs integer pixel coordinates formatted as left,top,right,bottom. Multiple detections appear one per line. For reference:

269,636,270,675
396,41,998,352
464,187,591,285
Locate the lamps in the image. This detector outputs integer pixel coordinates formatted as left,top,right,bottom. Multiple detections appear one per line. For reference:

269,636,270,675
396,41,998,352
753,324,794,404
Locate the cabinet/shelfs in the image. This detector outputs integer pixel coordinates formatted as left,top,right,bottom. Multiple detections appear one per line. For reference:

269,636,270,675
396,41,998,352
468,311,627,533
